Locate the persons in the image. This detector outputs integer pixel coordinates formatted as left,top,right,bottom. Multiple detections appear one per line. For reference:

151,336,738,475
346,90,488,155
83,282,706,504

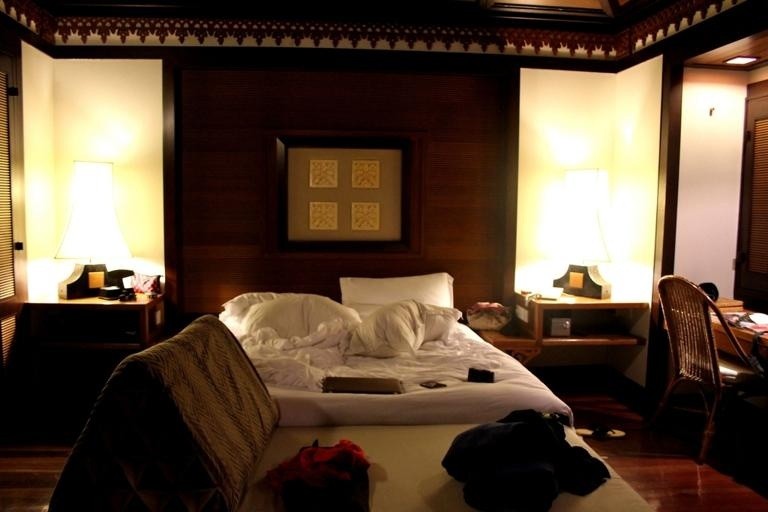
656,282,719,358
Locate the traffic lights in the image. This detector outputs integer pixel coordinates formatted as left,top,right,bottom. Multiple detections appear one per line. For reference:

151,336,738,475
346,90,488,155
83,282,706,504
340,271,454,310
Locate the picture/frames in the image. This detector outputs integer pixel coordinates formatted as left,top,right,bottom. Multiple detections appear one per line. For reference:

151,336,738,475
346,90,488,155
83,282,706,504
474,285,651,413
651,289,767,381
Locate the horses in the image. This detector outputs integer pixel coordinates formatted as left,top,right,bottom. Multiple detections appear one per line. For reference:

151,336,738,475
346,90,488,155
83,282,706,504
420,380,446,389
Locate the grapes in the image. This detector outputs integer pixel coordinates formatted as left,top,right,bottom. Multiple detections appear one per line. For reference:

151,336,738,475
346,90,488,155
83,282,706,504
25,291,167,433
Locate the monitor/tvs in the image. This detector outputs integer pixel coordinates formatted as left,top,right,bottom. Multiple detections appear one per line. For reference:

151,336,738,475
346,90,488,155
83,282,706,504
708,308,767,334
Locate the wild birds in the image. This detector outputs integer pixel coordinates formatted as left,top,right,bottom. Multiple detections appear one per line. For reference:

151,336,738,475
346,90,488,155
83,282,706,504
323,377,404,394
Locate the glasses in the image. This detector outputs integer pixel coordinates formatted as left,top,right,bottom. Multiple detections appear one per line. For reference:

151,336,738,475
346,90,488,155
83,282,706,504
554,225,611,300
60,224,127,298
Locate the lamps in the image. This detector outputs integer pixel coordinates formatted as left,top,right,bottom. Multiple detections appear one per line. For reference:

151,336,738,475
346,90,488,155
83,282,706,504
654,274,759,464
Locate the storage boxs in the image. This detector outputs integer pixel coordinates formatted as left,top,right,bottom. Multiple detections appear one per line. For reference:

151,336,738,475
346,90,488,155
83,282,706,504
46,315,653,512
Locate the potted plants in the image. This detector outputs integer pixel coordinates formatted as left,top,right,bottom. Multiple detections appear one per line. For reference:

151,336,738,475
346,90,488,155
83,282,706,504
267,126,424,258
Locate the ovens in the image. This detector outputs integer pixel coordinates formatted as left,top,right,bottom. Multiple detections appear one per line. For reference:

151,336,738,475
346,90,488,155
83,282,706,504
216,274,573,426
